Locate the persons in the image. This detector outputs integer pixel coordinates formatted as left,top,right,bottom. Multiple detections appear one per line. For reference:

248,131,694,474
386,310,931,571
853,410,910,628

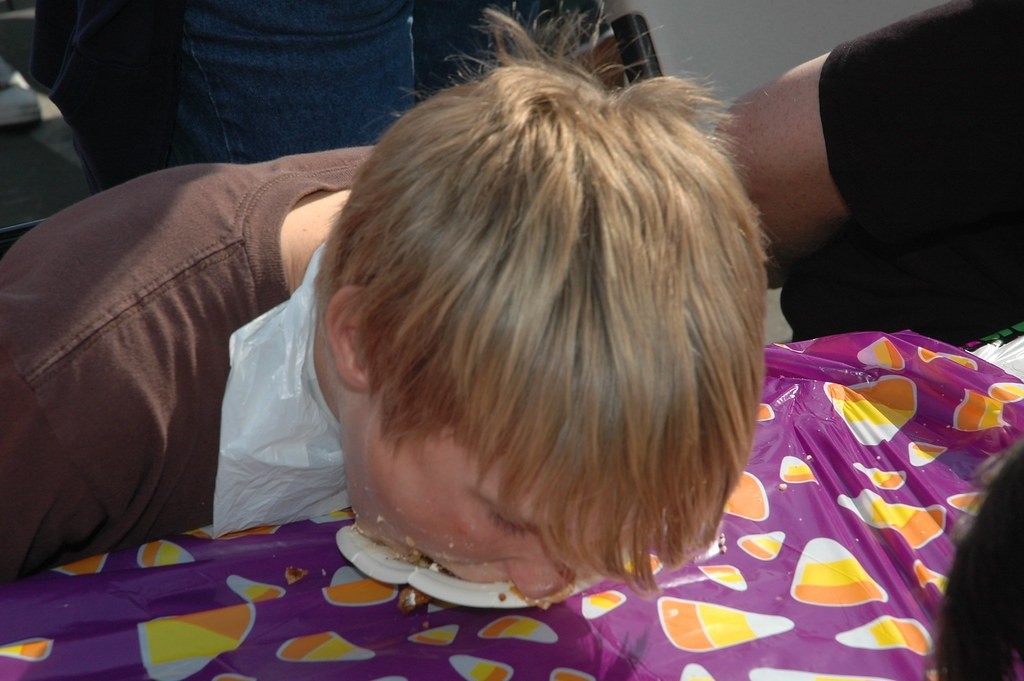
0,1,767,599
702,2,1024,680
